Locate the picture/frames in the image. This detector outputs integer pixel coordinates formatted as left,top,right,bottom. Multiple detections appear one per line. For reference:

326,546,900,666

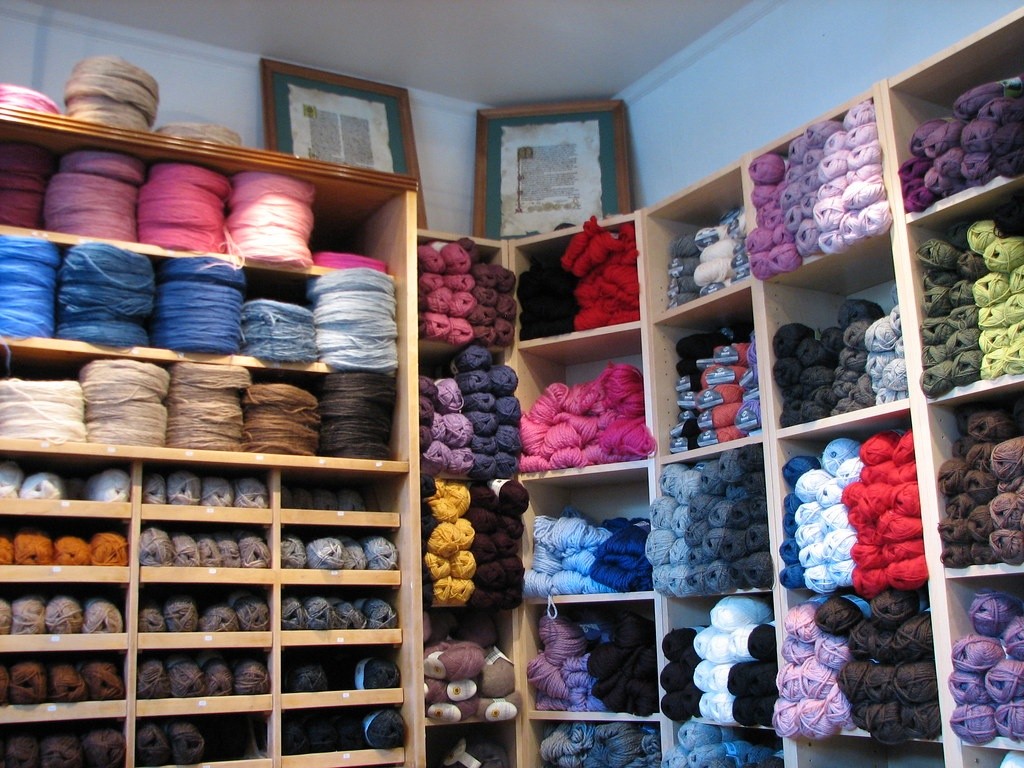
473,99,632,241
259,59,429,230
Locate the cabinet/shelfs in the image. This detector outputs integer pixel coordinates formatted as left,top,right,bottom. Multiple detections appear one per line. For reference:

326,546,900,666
0,5,1024,768
